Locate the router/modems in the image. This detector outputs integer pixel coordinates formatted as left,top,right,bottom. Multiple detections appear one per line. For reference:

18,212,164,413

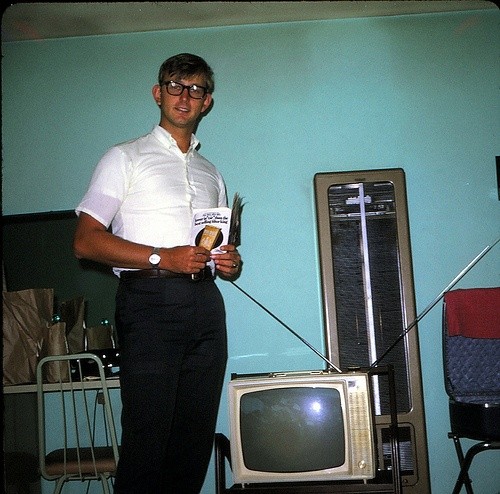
63,301,121,377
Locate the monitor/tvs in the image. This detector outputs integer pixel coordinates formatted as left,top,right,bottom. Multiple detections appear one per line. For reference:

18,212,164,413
228,370,376,483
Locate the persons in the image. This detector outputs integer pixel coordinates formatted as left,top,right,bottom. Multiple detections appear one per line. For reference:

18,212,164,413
71,53,241,494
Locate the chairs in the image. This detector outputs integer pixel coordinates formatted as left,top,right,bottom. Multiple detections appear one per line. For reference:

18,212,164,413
216,365,401,494
36,353,120,494
442,287,500,493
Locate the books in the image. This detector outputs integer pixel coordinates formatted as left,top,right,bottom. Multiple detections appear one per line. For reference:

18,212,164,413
189,191,249,254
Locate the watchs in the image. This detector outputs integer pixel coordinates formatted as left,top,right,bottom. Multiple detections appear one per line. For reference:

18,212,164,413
148,247,161,269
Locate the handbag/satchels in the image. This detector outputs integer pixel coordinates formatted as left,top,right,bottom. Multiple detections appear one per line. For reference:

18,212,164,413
0,263,54,385
45,322,70,381
64,296,85,353
74,348,121,377
85,324,115,350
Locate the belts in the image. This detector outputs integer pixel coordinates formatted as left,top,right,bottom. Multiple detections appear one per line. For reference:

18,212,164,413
120,266,212,282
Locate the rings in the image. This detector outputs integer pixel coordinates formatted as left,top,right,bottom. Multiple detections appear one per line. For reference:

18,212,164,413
233,261,238,268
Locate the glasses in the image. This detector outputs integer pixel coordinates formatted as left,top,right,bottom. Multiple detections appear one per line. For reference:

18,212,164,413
160,80,208,100
